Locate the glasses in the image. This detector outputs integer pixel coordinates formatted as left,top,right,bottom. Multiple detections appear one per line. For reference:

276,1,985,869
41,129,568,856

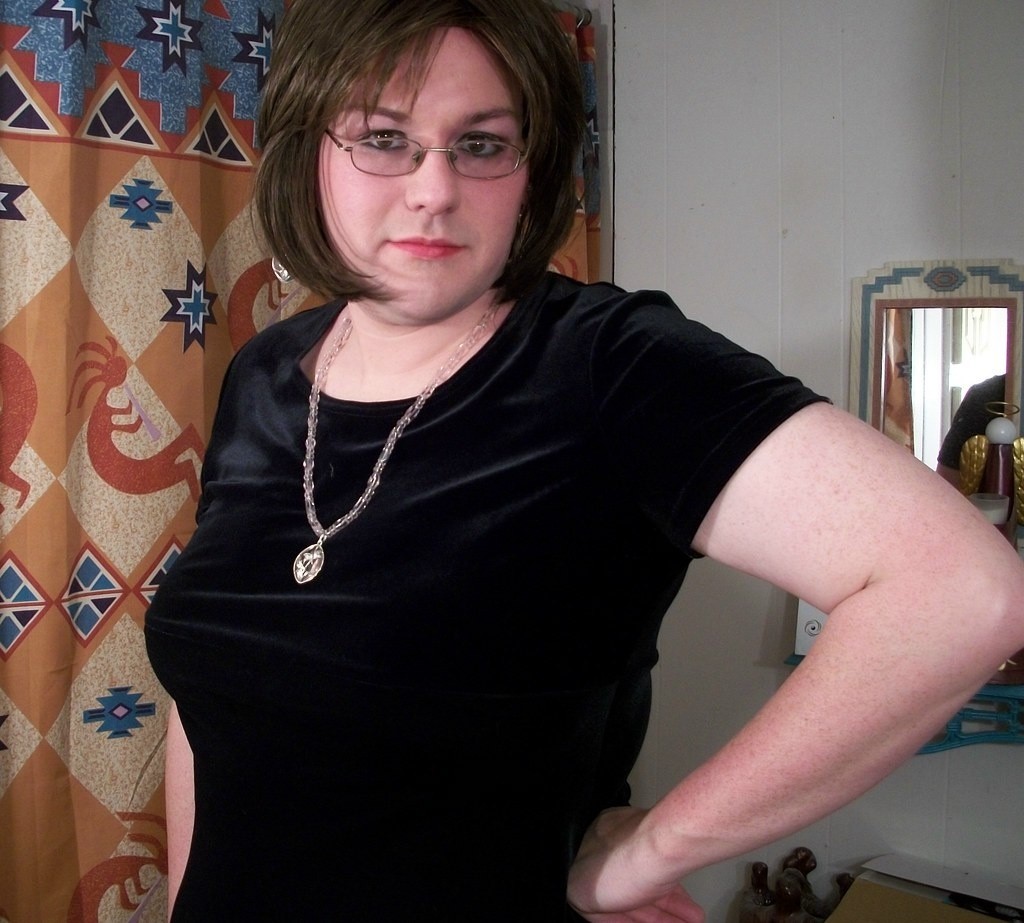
324,129,526,179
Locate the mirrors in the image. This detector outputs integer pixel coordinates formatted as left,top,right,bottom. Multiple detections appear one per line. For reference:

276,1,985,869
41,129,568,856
872,298,1011,496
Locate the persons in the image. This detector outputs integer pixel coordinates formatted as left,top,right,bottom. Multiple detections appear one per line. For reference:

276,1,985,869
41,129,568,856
143,0,1024,923
935,374,1005,487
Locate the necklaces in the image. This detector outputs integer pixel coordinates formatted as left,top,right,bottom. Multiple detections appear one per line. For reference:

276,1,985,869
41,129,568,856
290,298,501,585
